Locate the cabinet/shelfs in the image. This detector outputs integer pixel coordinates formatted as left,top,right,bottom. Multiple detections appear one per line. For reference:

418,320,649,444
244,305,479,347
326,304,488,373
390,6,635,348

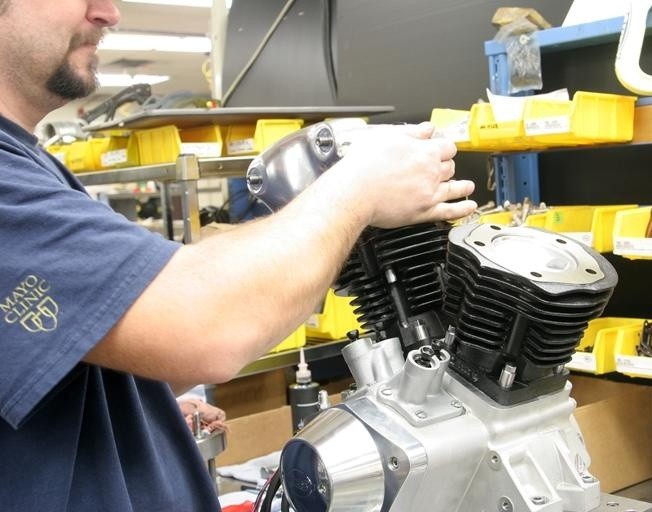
72,155,370,405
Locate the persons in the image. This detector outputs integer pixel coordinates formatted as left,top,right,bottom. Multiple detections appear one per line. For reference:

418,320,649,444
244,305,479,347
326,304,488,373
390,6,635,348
0,0,479,512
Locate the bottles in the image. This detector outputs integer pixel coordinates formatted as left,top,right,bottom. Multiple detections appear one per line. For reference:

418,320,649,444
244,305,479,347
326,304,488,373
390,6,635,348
287,347,321,437
